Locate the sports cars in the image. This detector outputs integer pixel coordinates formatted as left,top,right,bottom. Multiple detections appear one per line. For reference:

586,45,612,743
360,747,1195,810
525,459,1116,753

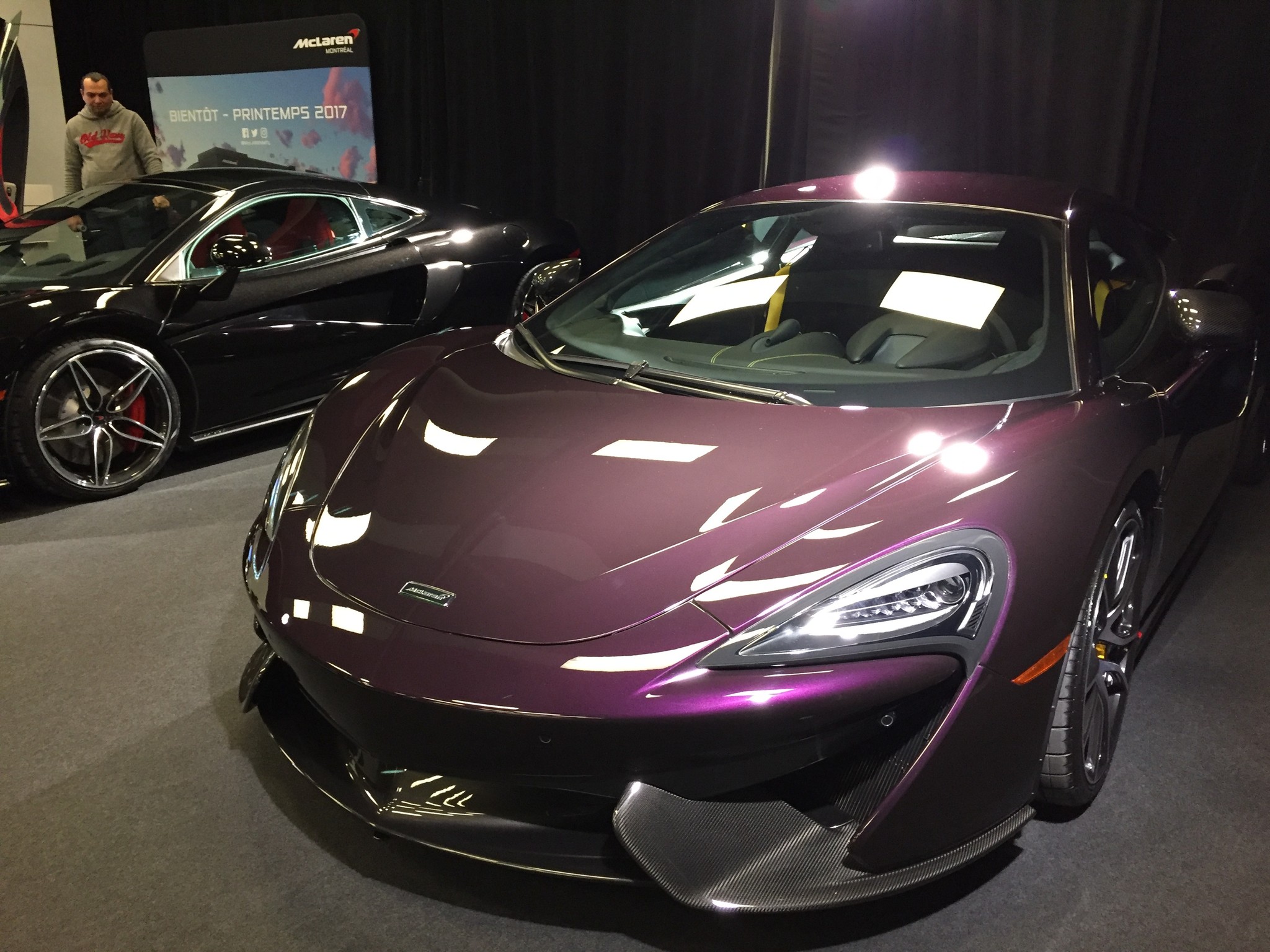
240,167,1269,946
0,163,593,501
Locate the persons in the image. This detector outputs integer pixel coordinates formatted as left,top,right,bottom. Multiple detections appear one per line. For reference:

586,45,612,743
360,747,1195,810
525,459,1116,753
64,71,172,260
